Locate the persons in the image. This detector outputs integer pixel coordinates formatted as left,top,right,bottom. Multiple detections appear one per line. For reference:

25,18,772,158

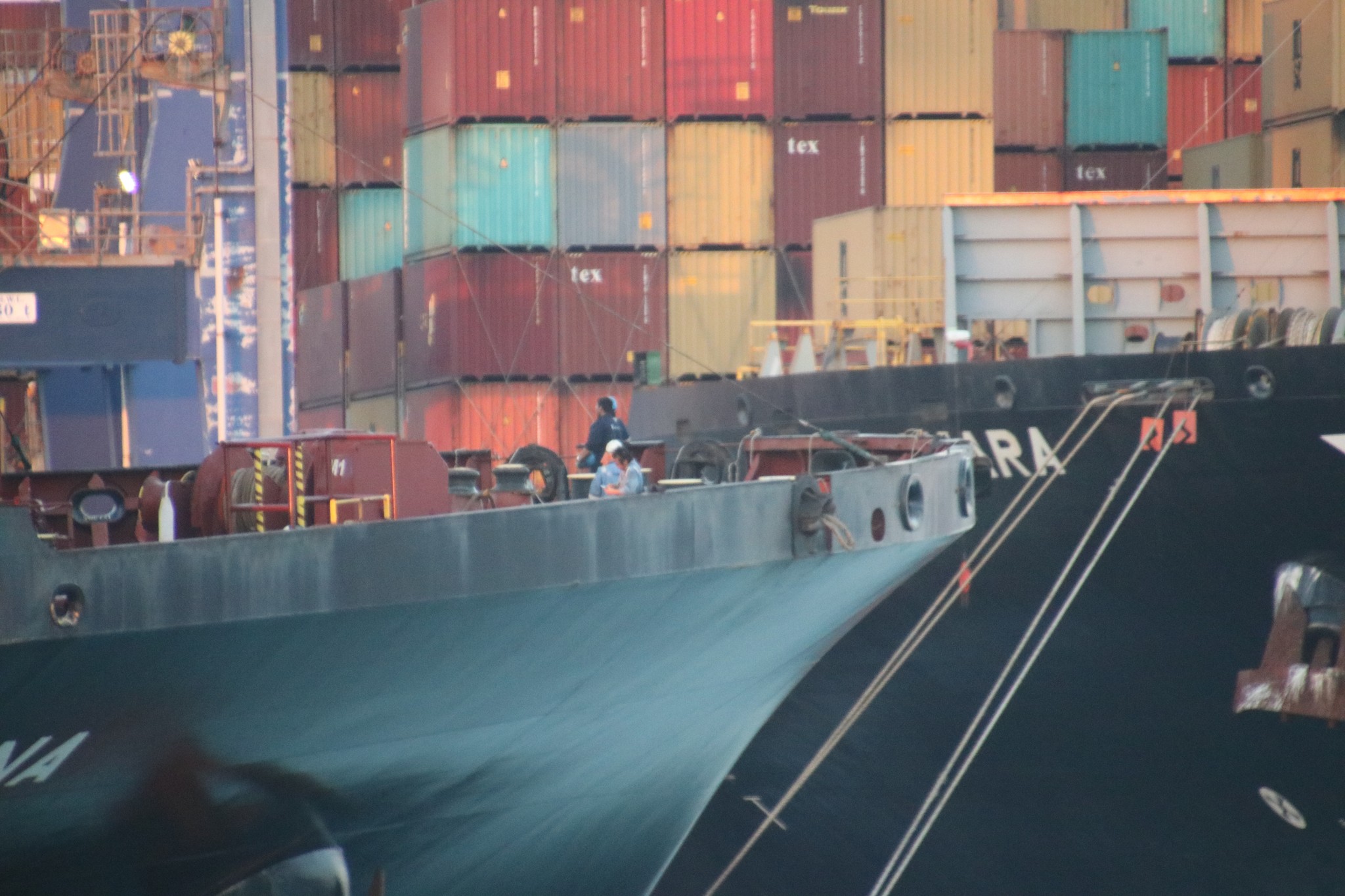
576,396,629,473
587,440,625,499
603,447,643,496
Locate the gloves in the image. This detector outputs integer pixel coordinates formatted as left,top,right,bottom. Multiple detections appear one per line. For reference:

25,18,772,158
575,456,581,467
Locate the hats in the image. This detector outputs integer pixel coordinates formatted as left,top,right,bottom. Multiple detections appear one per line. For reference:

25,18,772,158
597,396,617,412
605,439,624,453
